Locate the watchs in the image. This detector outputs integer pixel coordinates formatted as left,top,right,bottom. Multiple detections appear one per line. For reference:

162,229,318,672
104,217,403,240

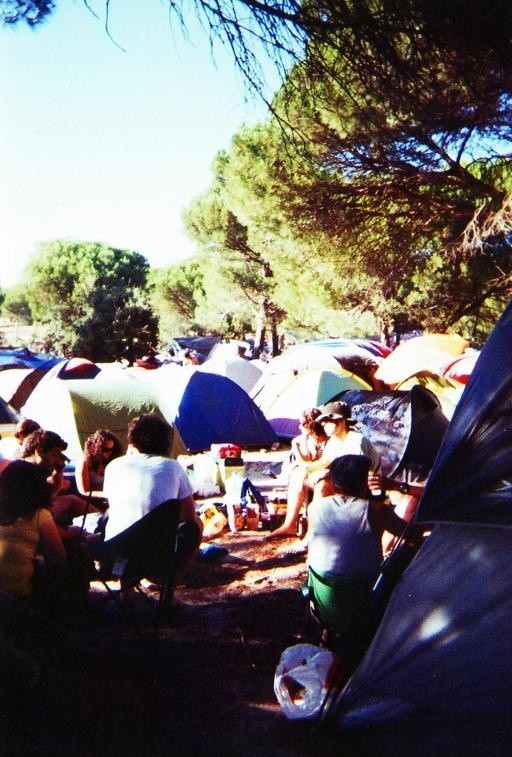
399,483,412,494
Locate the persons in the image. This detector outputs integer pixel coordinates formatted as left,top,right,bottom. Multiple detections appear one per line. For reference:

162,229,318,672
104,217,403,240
262,405,327,543
1,460,86,648
301,448,421,645
100,413,204,618
81,429,125,493
18,429,105,559
282,396,384,558
368,469,425,552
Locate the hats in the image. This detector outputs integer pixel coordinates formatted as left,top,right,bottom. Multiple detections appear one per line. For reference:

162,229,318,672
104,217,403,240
315,401,357,426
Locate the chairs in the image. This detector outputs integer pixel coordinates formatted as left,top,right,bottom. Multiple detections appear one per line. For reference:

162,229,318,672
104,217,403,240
91,498,181,620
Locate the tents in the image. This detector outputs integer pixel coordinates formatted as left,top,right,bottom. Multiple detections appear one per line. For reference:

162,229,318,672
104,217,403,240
323,295,512,757
0,330,486,482
